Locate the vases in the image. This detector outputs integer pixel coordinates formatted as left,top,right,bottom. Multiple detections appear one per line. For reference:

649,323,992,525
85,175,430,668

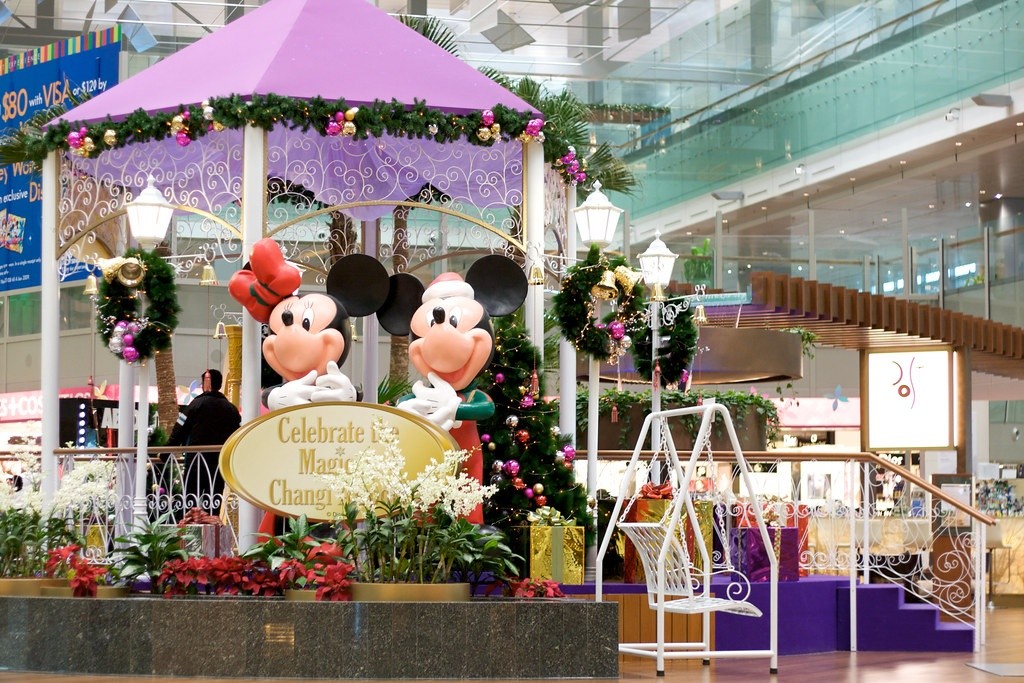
171,593,285,601
287,588,339,603
350,581,472,604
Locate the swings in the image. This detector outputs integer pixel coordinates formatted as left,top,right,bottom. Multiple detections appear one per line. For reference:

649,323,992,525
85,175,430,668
617,415,763,620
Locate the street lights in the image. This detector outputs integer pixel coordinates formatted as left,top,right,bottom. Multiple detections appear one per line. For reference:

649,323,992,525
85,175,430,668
123,172,177,592
636,230,679,486
569,180,622,590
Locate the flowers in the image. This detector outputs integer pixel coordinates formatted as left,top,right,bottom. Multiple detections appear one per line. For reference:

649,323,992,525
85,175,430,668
161,516,352,600
325,413,526,591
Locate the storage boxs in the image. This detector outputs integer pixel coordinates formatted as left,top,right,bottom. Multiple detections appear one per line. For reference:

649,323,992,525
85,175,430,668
528,525,585,586
727,529,799,583
625,498,713,587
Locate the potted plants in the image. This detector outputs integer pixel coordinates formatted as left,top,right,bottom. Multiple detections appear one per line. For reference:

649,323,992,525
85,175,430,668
0,500,203,598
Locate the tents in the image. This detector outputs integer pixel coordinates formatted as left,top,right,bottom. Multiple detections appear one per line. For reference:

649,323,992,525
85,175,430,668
39,0,545,576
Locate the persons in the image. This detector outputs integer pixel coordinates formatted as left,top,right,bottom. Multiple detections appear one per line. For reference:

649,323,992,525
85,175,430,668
157,369,242,525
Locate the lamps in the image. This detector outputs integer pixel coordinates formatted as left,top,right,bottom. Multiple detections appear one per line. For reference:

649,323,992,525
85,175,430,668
637,227,679,488
124,170,176,535
573,178,625,583
